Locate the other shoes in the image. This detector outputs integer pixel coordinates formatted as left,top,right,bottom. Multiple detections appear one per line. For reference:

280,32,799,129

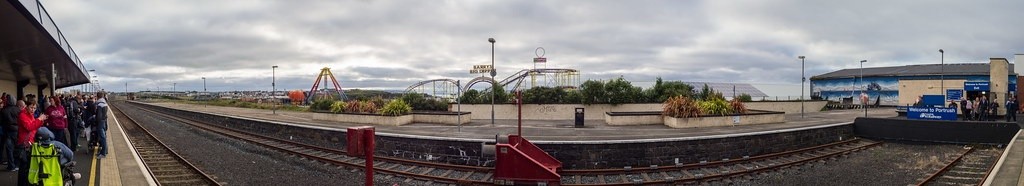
3,162,8,164
14,167,20,170
80,134,86,137
9,169,16,171
76,144,82,147
95,152,105,159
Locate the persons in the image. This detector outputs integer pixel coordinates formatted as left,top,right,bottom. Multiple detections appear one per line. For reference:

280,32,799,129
0,88,109,171
33,126,82,180
913,93,1019,122
16,99,47,186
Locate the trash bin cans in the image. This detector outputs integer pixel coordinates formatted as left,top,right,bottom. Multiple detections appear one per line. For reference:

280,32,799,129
574,107,585,127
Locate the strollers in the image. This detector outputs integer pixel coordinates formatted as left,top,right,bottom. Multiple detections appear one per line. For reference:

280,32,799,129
28,140,75,186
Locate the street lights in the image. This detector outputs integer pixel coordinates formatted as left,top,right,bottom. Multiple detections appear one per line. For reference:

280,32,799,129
272,66,278,114
799,56,806,118
81,69,102,95
860,59,868,112
173,83,176,104
202,77,206,108
489,37,496,124
939,49,944,95
